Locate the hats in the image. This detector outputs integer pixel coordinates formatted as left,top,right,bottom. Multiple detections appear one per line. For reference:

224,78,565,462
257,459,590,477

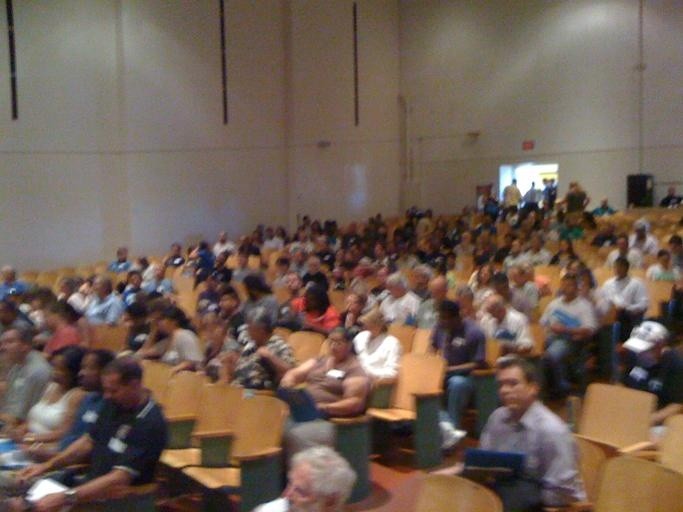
622,321,669,353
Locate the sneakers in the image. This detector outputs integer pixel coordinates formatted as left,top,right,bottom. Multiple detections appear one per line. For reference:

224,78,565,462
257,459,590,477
441,430,467,449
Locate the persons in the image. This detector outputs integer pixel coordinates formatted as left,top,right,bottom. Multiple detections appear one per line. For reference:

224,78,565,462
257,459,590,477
1,178,682,510
